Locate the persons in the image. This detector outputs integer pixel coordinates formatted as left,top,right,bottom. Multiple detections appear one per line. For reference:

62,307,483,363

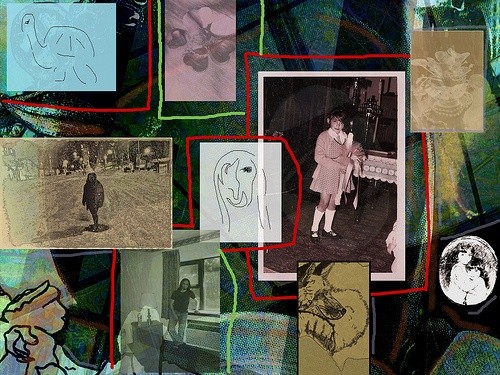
167,278,200,348
447,241,491,305
82,173,104,232
309,109,355,243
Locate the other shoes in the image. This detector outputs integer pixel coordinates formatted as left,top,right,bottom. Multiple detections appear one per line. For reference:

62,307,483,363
310,227,320,244
322,228,343,241
